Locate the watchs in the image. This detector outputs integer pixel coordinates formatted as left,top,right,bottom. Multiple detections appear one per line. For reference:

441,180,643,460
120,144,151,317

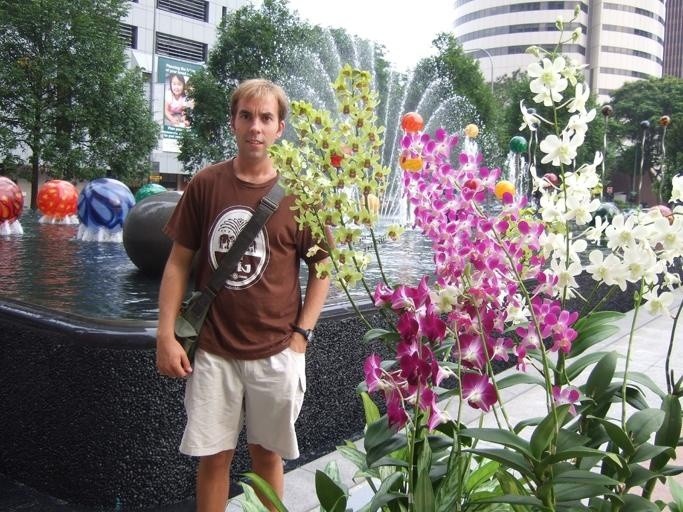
287,321,315,349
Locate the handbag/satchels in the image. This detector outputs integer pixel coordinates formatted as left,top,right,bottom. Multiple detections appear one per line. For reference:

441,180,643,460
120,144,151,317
166,288,211,382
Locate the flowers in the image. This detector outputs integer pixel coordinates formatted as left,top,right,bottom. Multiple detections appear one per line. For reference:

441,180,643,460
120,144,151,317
236,5,683,512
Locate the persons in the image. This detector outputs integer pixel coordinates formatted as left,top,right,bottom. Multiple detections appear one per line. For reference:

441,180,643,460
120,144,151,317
154,77,330,512
164,73,187,127
